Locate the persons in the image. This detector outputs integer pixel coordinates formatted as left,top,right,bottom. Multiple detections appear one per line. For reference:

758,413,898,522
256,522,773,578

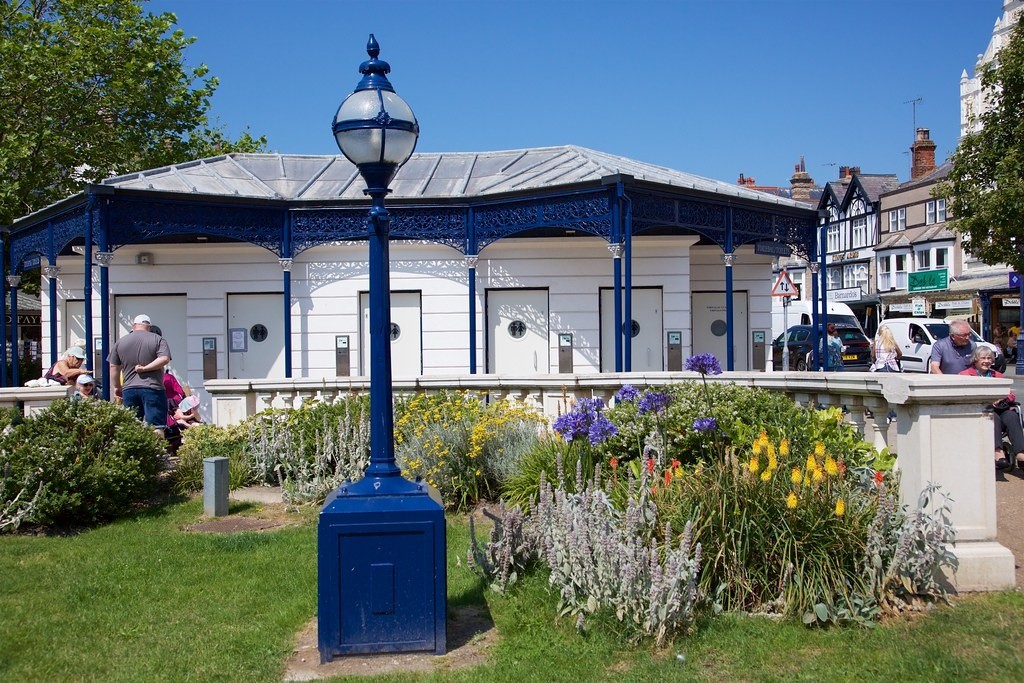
914,328,928,343
993,321,1023,363
44,314,202,440
931,320,977,374
871,324,902,372
958,346,1024,468
819,324,847,372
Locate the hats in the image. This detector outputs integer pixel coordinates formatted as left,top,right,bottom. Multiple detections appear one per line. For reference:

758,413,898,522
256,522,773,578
133,314,153,326
77,374,96,385
67,346,86,358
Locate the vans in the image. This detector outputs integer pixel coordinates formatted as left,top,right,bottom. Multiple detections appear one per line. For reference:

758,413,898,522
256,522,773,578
875,318,1007,375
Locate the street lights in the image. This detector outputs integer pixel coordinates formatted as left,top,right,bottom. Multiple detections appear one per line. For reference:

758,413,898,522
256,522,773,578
315,31,449,665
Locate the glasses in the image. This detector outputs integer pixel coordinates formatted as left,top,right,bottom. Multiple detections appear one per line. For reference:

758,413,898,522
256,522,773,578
954,333,971,339
74,356,84,361
79,383,95,387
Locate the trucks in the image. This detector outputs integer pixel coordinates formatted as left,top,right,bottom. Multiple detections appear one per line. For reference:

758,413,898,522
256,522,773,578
771,301,871,346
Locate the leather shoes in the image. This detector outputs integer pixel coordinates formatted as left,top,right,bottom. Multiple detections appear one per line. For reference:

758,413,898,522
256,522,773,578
995,458,1008,466
1017,460,1024,467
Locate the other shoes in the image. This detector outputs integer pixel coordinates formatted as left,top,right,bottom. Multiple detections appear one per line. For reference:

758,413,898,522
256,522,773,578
887,416,897,424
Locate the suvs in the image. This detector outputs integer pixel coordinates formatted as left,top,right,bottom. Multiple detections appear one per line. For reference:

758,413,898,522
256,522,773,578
771,326,871,372
